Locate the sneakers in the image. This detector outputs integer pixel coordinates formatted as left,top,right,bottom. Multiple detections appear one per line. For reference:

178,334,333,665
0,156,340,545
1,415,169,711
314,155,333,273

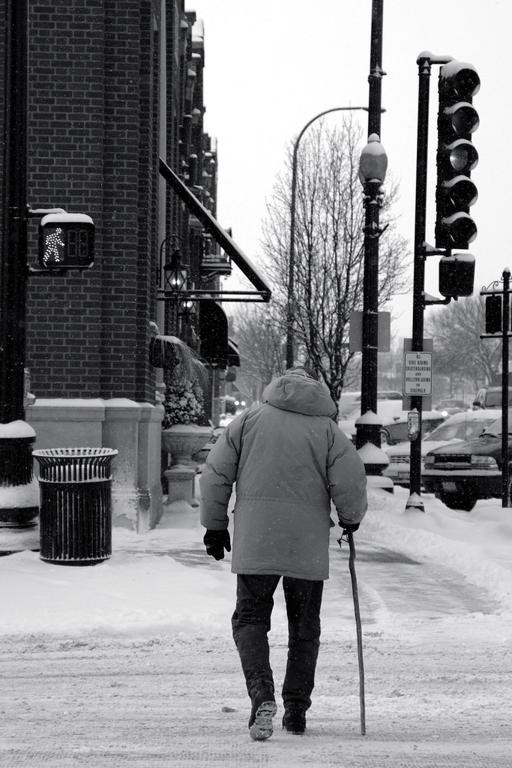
249,693,277,740
282,711,306,734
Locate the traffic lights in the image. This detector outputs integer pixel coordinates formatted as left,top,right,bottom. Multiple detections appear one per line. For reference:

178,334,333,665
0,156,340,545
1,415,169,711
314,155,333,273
36,211,95,269
436,61,481,300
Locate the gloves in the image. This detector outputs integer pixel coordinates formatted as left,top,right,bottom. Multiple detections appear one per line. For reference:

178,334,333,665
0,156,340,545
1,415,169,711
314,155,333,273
204,530,231,560
338,521,360,534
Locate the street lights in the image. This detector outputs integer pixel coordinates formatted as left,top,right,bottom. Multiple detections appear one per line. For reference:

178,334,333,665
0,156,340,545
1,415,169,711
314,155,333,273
355,131,392,492
283,103,389,377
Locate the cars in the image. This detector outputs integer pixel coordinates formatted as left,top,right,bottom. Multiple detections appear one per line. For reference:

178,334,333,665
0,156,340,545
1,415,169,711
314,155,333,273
381,406,508,492
419,406,512,512
471,384,512,412
337,399,453,448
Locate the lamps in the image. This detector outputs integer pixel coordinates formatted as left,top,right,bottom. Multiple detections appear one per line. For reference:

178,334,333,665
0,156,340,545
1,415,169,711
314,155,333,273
159,235,189,299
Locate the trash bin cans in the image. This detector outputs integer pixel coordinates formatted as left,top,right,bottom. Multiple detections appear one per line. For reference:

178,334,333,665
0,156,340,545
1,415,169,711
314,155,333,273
32,447,118,567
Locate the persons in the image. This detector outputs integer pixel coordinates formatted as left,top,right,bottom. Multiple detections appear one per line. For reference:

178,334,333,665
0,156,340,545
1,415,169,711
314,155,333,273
199,366,368,740
473,401,482,410
